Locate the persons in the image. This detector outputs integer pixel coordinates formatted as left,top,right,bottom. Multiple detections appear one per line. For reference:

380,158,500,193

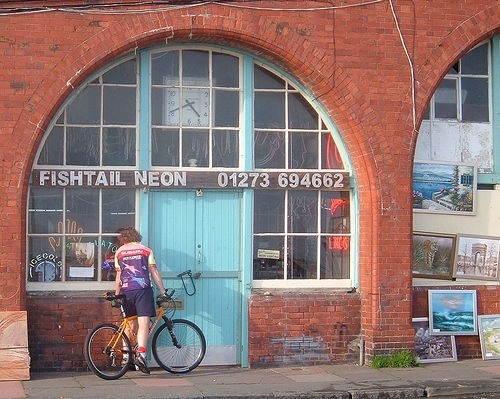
113,227,169,374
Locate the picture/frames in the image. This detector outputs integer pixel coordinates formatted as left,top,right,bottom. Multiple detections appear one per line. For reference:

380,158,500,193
428,289,479,336
413,231,456,280
452,233,500,282
412,159,478,216
478,314,500,361
411,317,458,364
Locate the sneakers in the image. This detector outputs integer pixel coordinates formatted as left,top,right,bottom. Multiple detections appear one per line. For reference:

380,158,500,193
121,358,136,371
135,356,150,374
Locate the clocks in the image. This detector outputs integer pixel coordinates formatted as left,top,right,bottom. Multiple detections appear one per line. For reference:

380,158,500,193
164,82,212,128
34,261,56,281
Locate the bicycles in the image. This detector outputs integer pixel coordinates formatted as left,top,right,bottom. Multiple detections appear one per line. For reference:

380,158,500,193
82,288,206,380
